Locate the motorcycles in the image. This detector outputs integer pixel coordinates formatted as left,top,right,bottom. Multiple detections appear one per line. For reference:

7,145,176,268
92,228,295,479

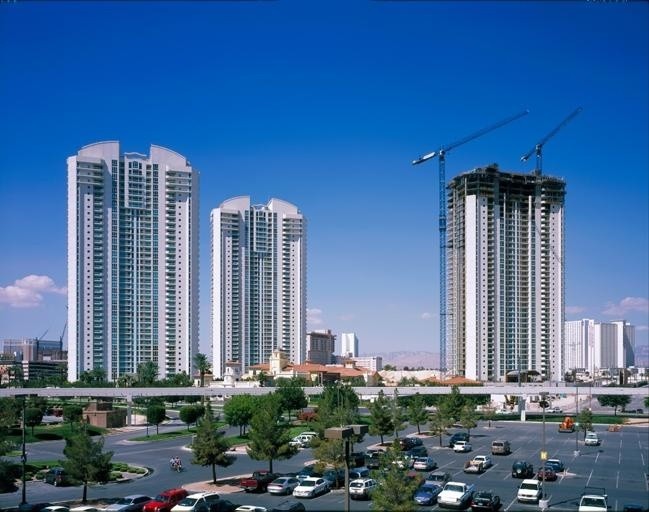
170,462,182,473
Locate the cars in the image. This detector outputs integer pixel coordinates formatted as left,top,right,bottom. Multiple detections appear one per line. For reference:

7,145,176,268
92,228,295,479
495,409,513,415
424,471,451,489
413,483,442,506
293,477,329,498
472,455,491,469
366,436,428,471
538,466,557,481
70,506,105,512
413,456,437,471
288,432,320,448
453,441,472,453
584,433,600,446
105,495,155,511
296,464,327,480
470,491,500,511
329,467,371,489
576,493,613,512
40,505,69,512
548,408,562,413
544,459,564,473
623,504,645,512
268,477,300,495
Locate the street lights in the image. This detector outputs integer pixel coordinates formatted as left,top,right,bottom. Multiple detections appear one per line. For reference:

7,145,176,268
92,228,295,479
11,392,39,508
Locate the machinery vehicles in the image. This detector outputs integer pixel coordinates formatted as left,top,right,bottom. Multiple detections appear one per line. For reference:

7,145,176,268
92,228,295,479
557,416,576,433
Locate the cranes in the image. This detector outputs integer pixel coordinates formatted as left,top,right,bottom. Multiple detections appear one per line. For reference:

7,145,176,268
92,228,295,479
57,319,69,361
413,106,528,381
520,107,602,175
34,328,49,360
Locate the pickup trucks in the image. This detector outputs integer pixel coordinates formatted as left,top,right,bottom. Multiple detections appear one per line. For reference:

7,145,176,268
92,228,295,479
437,482,475,509
517,479,543,504
240,470,276,492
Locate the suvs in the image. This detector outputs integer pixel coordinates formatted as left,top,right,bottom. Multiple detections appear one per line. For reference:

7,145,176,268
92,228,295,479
144,488,188,512
449,433,469,448
44,468,68,487
233,505,267,512
268,501,305,512
348,479,378,500
170,492,225,512
512,461,533,479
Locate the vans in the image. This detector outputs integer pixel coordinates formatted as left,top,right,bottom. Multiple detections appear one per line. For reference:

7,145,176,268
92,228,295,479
491,440,511,456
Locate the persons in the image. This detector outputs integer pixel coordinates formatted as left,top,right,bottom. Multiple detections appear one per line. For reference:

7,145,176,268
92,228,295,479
170,456,181,473
